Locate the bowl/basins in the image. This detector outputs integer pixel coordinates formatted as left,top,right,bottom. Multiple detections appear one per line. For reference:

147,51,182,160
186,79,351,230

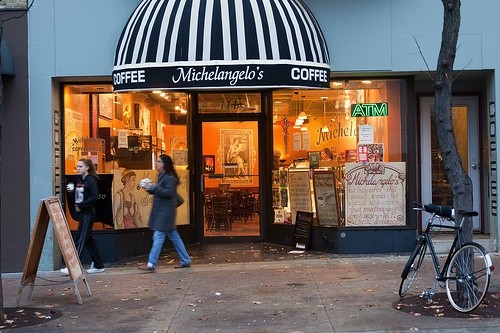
141,178,151,187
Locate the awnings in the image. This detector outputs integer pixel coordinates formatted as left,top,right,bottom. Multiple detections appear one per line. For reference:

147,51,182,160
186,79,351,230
111,0,332,93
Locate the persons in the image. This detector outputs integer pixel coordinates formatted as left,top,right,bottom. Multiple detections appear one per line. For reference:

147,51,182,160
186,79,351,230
274,149,281,170
59,158,106,274
139,154,192,272
320,148,335,171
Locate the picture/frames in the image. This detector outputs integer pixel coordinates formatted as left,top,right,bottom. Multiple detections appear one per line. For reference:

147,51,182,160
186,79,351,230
204,155,215,174
220,129,253,183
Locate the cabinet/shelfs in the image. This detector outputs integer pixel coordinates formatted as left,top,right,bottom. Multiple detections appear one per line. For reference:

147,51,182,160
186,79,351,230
273,169,288,208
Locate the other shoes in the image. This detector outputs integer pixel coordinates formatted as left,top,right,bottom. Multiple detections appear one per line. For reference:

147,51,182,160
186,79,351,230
174,263,191,269
139,262,154,271
59,267,71,275
86,261,105,275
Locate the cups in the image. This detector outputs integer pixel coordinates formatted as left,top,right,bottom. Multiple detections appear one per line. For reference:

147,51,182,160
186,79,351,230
68,182,74,192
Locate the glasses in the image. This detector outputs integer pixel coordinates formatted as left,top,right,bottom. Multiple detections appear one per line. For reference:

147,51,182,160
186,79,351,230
156,161,163,163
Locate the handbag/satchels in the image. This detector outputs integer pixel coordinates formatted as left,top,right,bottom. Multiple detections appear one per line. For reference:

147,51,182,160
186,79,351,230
175,192,185,208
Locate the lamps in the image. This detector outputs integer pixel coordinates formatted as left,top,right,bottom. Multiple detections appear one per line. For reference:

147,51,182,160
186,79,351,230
294,92,329,132
221,100,245,110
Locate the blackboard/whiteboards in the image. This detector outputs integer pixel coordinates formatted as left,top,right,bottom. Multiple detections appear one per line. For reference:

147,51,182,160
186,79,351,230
18,196,86,284
291,211,314,252
288,170,310,225
312,168,340,227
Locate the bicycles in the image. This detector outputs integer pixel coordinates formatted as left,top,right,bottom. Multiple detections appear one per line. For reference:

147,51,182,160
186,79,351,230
398,202,495,314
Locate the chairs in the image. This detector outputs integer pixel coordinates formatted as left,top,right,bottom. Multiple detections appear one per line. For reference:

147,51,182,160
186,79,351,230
204,184,258,232
